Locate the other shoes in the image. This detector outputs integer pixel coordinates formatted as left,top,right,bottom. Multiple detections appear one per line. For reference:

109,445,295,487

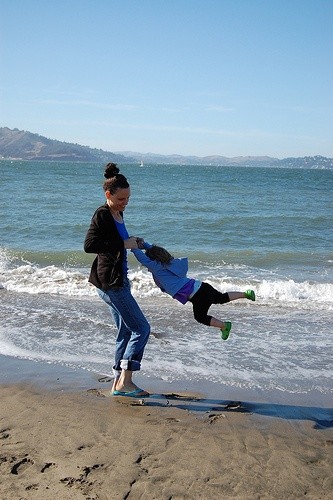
221,322,231,339
245,290,255,301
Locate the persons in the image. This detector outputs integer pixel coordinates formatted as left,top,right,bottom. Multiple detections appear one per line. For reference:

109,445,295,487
130,236,256,340
83,161,152,399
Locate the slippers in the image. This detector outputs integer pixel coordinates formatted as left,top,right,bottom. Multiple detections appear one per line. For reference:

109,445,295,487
113,383,149,398
